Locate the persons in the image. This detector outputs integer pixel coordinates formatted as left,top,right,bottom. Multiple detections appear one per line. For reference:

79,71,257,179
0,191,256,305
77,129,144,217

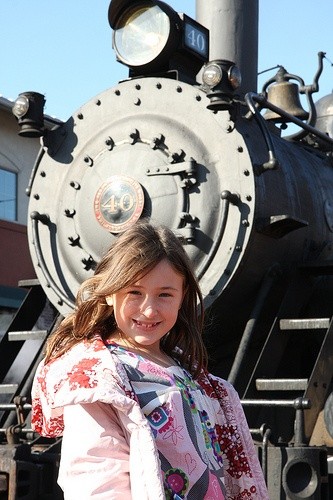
29,222,269,500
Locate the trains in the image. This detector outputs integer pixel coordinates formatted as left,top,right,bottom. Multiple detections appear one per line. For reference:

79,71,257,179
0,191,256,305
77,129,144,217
1,0,333,500
